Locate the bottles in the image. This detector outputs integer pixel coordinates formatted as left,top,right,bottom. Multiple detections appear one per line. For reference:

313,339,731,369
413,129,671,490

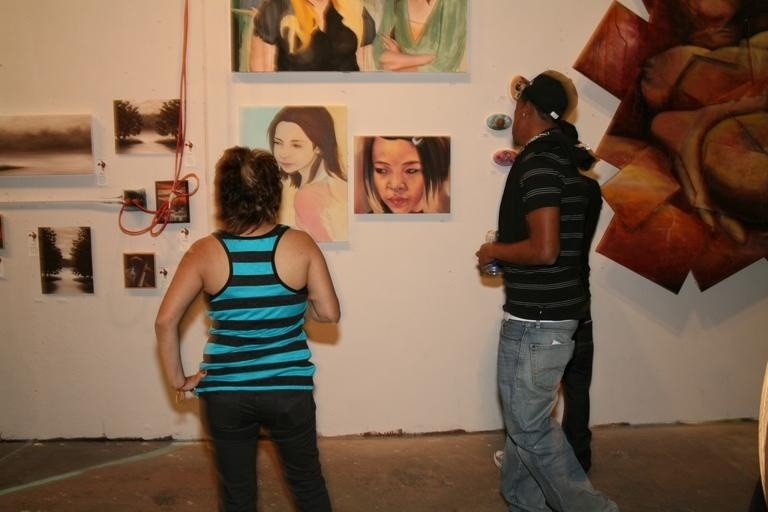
481,230,503,287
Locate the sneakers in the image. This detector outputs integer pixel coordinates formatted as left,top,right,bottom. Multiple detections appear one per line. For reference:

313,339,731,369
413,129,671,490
494,450,505,470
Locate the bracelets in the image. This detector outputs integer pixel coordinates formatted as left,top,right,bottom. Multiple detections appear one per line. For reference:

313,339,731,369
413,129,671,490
173,377,187,407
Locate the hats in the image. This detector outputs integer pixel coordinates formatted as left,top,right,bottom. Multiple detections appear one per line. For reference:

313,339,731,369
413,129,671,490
522,73,567,122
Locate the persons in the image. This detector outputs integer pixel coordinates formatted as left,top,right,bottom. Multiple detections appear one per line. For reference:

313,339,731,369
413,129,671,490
249,0,376,72
493,121,594,475
154,144,342,512
475,73,619,512
371,0,467,72
266,106,348,243
360,136,450,214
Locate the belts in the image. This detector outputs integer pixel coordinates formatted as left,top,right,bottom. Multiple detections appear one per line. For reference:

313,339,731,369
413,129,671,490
503,312,572,323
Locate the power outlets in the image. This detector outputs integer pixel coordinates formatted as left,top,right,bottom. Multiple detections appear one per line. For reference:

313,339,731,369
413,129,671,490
122,190,146,211
